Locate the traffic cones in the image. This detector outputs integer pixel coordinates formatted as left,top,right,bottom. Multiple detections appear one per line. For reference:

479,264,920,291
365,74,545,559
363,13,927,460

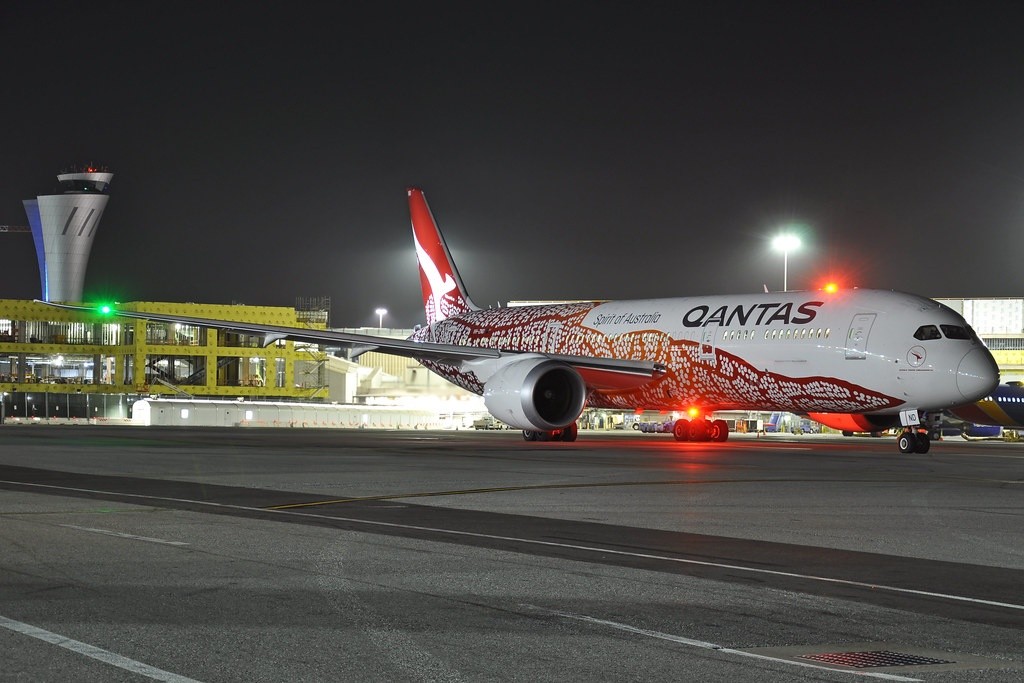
763,429,767,435
757,431,759,438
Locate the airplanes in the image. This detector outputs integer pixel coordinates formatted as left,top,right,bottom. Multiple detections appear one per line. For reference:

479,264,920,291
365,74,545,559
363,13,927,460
34,188,1000,456
791,380,1024,439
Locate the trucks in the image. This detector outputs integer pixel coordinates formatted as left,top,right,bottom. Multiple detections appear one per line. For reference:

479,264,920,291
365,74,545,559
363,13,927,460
474,416,503,430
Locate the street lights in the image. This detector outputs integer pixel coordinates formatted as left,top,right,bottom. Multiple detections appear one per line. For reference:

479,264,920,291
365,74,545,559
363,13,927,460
375,308,388,328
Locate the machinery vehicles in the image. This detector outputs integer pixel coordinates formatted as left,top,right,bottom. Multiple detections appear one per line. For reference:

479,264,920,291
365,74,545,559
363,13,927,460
631,418,640,430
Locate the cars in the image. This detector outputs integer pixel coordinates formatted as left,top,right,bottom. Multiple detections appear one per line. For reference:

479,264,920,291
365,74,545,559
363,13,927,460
791,419,814,434
